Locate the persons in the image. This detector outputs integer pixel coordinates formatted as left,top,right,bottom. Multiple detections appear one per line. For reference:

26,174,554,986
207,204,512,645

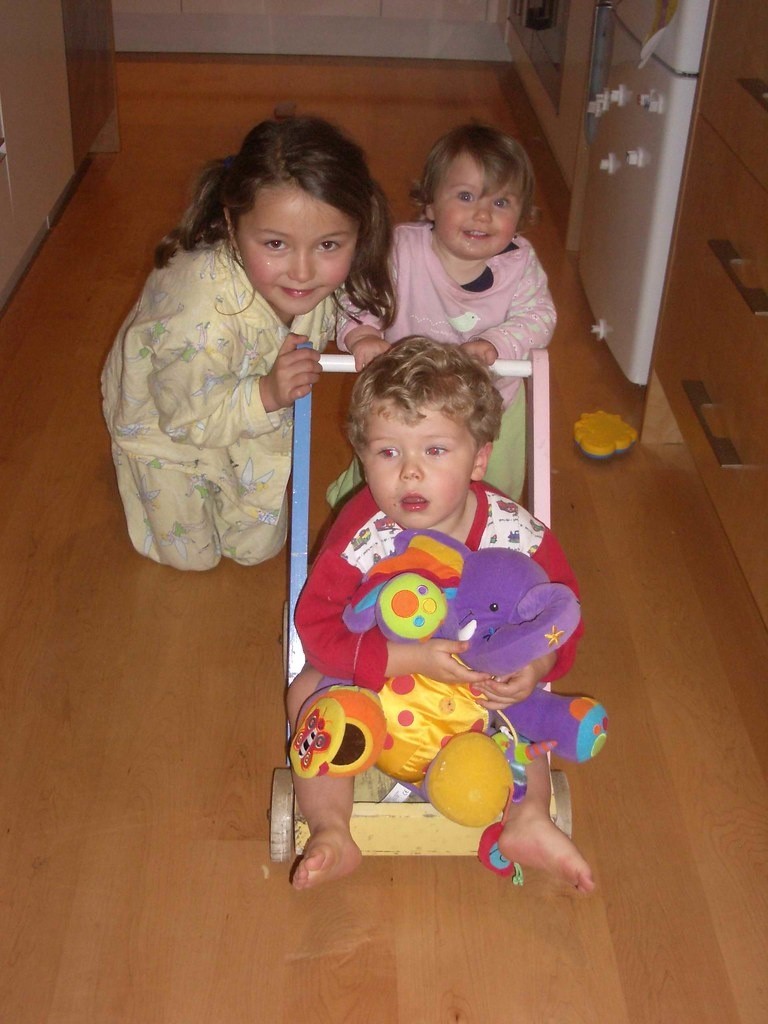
327,123,556,517
100,117,398,572
286,335,595,892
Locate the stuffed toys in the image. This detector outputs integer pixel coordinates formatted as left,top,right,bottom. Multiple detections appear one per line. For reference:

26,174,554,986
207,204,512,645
289,528,609,826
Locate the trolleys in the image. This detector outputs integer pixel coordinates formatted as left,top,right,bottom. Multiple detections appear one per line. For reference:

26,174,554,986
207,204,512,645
265,339,574,865
